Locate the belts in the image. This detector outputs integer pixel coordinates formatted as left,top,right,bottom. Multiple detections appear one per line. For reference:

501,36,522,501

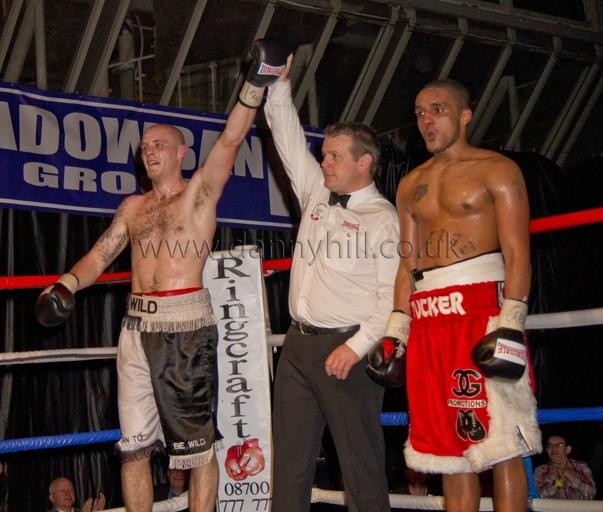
291,320,359,336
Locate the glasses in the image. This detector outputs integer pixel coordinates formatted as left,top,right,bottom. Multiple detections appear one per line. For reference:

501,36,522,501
543,441,569,452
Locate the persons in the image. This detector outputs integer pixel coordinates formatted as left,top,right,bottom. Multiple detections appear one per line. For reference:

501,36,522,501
154,462,188,502
43,478,106,512
36,38,275,512
259,49,404,512
363,79,542,512
389,462,441,497
535,431,598,498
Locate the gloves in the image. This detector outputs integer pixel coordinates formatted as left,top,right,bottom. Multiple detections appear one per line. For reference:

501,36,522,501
35,274,79,329
224,439,265,481
456,409,487,442
239,38,289,109
472,298,530,380
365,308,412,388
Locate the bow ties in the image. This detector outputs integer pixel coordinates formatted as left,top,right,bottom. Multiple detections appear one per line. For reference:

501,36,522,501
328,192,351,208
